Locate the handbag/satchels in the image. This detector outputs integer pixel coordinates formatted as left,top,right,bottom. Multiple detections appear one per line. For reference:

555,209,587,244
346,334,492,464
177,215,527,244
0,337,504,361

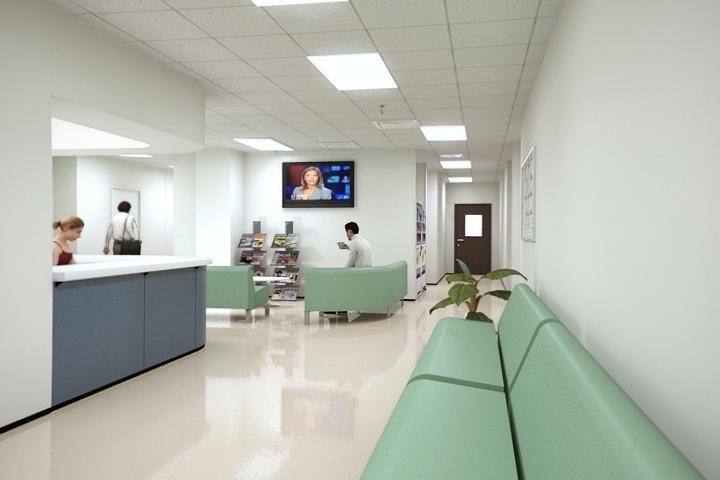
121,238,143,257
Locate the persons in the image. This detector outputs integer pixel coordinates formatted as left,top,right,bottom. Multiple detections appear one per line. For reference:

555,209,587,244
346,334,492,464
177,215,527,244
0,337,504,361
52,216,86,265
103,201,142,254
292,167,331,200
323,222,374,315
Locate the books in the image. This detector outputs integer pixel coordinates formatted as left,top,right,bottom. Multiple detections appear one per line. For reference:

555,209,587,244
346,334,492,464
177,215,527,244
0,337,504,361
417,203,426,277
239,232,300,300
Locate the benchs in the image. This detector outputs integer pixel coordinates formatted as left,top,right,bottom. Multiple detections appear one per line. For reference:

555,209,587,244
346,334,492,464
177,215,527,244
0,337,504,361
362,323,708,480
206,265,271,322
356,281,564,390
303,259,408,324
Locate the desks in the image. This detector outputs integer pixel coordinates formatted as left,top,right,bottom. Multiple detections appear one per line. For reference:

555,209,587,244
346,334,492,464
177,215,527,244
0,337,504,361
251,273,292,309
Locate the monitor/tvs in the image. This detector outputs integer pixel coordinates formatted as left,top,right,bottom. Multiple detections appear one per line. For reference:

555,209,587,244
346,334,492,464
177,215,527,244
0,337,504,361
282,161,354,208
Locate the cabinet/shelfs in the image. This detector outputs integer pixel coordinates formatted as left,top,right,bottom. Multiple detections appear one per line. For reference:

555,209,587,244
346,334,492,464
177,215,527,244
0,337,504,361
235,220,268,277
271,219,302,302
